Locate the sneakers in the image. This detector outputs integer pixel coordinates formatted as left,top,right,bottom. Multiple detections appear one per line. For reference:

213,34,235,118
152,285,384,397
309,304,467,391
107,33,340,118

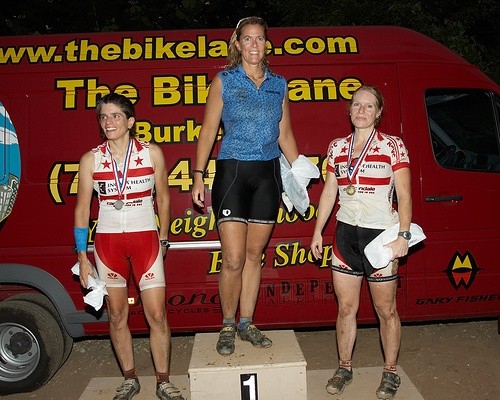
156,383,185,400
237,325,273,349
326,367,353,395
216,323,235,355
376,372,400,400
113,377,141,400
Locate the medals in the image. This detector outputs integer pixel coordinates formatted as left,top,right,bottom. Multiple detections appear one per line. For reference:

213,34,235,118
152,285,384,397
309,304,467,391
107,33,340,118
346,185,355,195
114,200,124,210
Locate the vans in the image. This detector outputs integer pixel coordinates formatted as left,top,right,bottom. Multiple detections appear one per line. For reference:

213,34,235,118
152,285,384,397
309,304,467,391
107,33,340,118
0,23,500,396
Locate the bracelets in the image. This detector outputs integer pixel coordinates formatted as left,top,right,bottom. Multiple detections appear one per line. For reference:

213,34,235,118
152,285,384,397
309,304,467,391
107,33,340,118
192,169,203,173
73,227,89,253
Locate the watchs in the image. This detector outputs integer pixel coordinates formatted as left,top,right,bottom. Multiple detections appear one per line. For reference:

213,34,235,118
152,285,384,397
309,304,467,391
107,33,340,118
159,240,169,246
398,231,411,240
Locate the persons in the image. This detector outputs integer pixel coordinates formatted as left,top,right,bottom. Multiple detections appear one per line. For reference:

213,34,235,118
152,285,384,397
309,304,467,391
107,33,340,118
310,85,411,399
191,17,298,356
73,93,184,400
